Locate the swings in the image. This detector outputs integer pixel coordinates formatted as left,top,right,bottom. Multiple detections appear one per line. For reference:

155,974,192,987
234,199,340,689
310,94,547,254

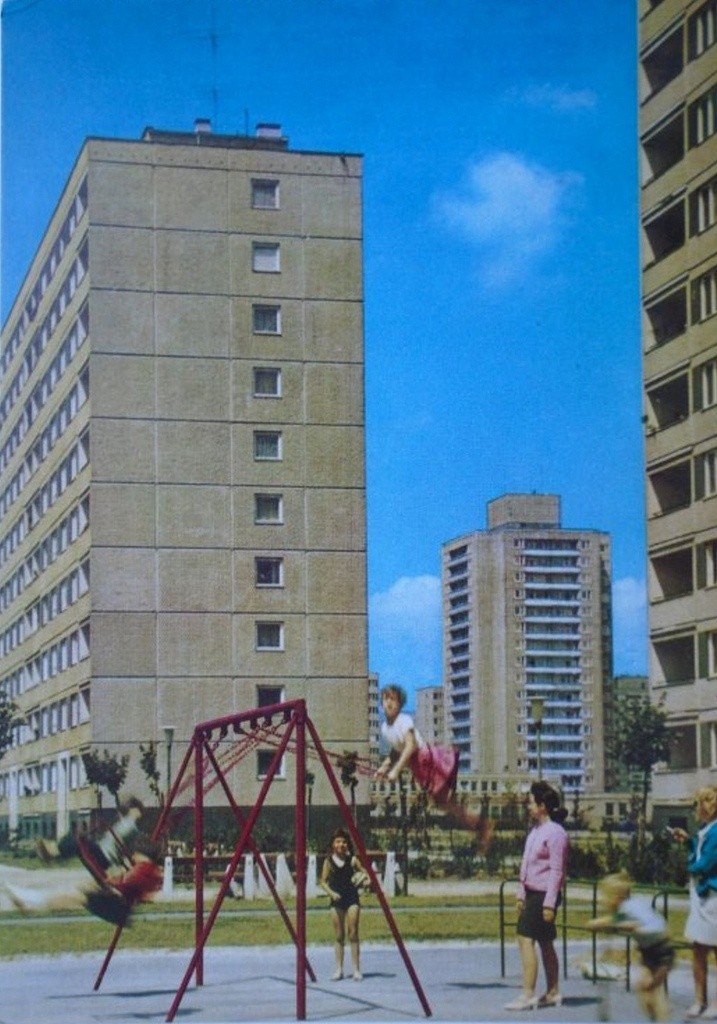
233,722,461,805
80,718,273,926
75,725,228,887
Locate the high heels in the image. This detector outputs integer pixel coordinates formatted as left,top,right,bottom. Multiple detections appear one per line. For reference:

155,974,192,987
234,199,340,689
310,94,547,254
505,996,538,1010
540,994,562,1008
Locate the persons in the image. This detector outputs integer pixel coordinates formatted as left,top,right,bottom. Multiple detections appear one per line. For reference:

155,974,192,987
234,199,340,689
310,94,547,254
8,798,162,921
504,783,569,1011
375,686,495,862
322,832,370,980
673,784,717,1019
587,874,676,1021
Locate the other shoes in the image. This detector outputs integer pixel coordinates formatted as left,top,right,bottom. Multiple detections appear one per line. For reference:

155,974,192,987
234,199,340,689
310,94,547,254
328,970,343,981
353,971,363,981
702,1006,717,1018
688,1003,705,1017
